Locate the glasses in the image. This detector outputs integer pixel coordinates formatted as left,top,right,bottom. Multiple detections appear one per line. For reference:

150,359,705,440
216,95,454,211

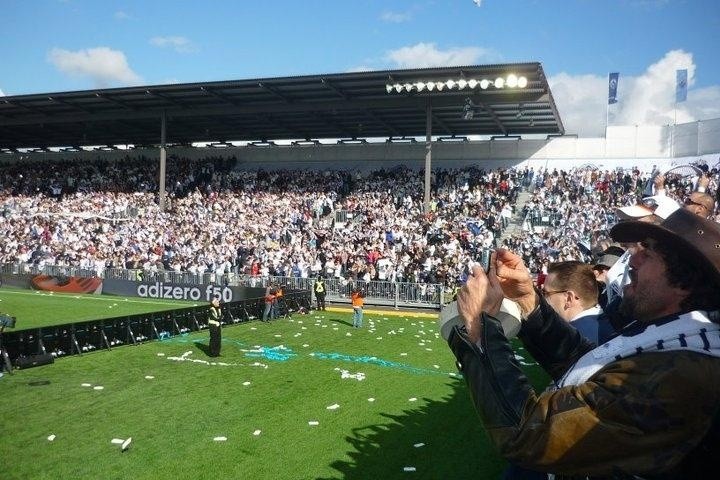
541,288,579,300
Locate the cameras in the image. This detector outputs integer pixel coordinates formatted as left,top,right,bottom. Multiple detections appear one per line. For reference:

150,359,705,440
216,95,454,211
481,249,496,274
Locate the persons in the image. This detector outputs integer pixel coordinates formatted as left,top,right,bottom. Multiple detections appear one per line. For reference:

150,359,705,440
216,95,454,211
435,152,719,480
1,153,534,304
314,275,327,311
352,287,366,328
264,282,275,321
208,298,224,357
273,283,290,317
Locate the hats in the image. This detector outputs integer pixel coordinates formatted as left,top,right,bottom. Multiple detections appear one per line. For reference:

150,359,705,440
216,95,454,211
592,192,720,273
